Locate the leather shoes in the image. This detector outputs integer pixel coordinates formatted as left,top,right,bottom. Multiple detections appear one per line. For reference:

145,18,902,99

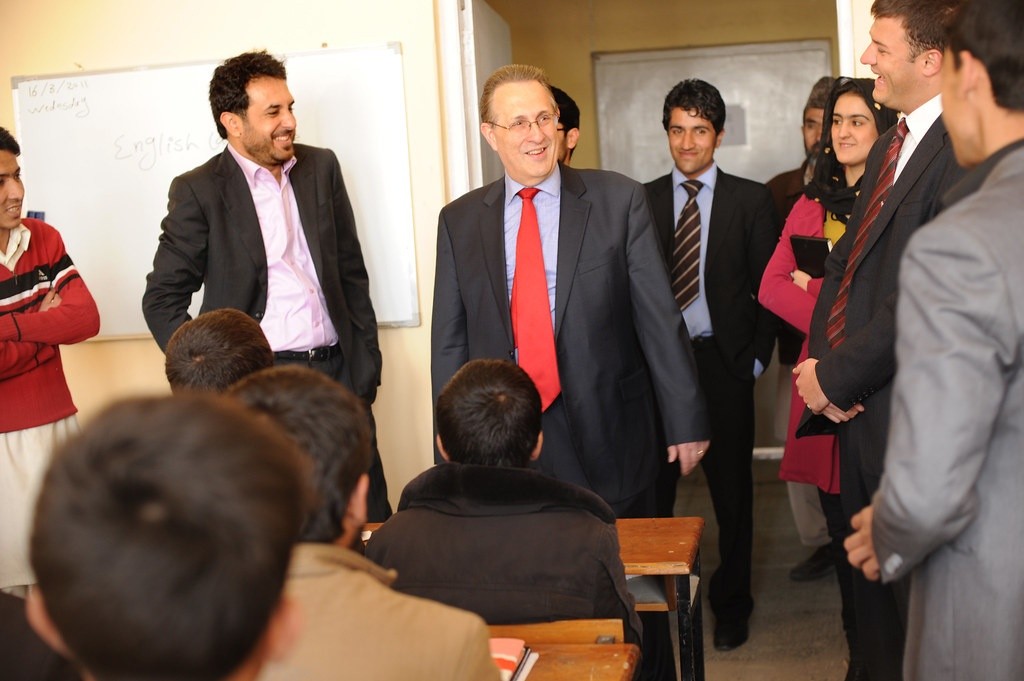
714,618,747,650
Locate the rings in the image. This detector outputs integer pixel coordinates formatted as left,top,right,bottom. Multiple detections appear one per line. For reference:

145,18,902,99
807,405,811,408
698,451,704,455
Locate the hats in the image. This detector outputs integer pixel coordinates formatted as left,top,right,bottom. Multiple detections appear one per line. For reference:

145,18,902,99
803,76,836,110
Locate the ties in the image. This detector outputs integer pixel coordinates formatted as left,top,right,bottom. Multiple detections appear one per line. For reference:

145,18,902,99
672,179,704,313
510,186,561,412
825,119,910,348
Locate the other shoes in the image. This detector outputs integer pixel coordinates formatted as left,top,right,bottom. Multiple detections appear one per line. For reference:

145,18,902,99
791,545,839,580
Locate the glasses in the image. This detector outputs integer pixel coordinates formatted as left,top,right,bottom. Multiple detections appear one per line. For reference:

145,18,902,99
488,113,555,136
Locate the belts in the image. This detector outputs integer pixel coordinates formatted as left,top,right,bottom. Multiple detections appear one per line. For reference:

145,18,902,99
273,345,340,361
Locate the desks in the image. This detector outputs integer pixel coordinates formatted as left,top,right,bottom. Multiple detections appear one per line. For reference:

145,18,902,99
360,516,705,681
517,644,644,680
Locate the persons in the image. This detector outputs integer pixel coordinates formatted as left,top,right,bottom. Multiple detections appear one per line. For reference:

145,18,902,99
431,69,714,519
140,50,391,523
0,126,101,598
0,306,652,681
791,0,965,681
766,74,900,582
639,76,783,652
842,0,1024,681
